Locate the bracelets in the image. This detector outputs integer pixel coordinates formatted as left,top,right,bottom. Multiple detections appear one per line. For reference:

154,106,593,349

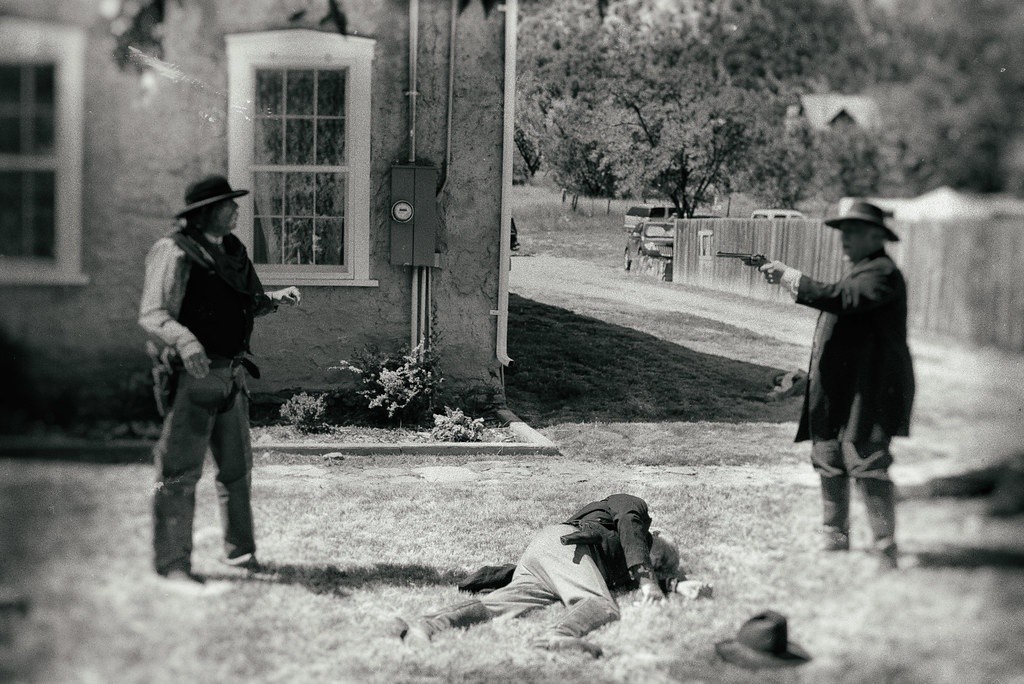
669,577,680,593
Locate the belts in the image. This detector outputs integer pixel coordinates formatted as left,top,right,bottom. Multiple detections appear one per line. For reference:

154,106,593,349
211,354,261,381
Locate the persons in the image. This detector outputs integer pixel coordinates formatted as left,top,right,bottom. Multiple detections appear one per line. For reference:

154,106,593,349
388,492,710,658
138,174,300,582
757,200,917,567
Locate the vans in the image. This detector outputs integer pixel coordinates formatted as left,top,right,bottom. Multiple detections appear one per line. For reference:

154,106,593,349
624,204,684,232
751,209,803,219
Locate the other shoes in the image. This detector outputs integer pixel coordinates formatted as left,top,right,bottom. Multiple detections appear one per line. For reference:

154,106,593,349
217,548,263,574
164,569,203,594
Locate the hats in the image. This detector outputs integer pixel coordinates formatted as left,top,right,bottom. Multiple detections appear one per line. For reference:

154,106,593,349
824,201,902,243
714,608,813,671
172,173,249,220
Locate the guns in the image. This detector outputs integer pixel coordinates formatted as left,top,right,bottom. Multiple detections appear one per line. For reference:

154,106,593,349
559,520,607,547
716,249,772,285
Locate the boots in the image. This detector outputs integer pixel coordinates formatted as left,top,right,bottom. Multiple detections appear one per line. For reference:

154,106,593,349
388,596,490,654
816,477,850,553
530,597,613,660
857,479,897,567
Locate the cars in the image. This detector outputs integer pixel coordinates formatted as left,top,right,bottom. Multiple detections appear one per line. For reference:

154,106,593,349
623,222,674,270
